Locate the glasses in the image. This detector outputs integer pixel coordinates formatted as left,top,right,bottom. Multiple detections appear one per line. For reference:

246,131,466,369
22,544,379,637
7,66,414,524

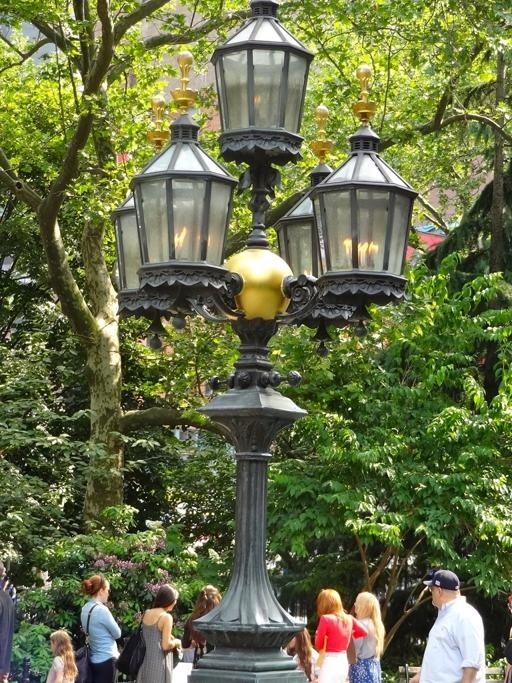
428,585,433,591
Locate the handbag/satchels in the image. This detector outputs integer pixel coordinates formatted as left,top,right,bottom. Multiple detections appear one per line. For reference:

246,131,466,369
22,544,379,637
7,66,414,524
115,631,146,679
74,645,93,683
347,637,357,664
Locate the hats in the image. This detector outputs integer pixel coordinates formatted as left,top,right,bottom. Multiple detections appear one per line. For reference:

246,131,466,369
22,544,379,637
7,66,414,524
423,570,460,590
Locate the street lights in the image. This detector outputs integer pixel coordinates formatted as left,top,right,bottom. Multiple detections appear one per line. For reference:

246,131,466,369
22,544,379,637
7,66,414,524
106,0,421,683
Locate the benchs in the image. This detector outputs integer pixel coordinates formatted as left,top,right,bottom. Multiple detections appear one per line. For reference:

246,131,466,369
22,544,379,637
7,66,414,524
397,662,503,683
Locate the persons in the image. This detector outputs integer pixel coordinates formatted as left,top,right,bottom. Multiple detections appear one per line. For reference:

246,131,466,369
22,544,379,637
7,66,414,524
408,569,486,682
81,574,123,682
0,560,16,683
314,588,352,683
348,591,386,683
502,594,512,683
133,585,180,683
284,627,319,682
180,584,224,669
45,630,79,682
